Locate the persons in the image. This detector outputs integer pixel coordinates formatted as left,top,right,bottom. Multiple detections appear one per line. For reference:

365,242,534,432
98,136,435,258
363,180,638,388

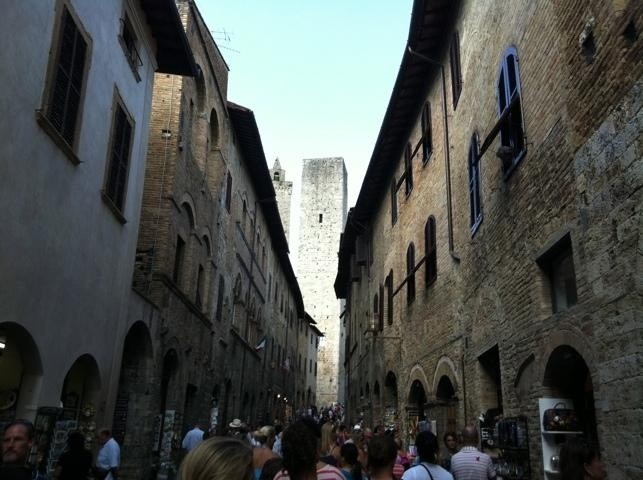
559,437,605,480
54,432,93,480
167,400,511,480
0,419,36,480
93,427,121,480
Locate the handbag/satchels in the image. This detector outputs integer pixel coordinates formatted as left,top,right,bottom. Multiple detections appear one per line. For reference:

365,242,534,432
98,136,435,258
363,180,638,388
544,408,579,431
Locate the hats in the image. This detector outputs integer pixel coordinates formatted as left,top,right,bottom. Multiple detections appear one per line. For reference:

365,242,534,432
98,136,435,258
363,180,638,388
229,418,242,428
